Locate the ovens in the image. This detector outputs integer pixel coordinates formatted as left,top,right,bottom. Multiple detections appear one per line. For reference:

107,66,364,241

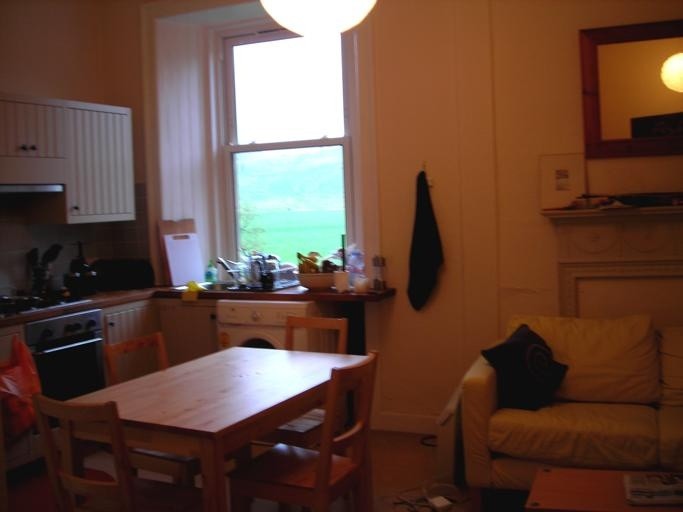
27,329,105,436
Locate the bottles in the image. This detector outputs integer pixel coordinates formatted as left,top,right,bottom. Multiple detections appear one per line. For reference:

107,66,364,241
205,259,217,282
371,255,386,292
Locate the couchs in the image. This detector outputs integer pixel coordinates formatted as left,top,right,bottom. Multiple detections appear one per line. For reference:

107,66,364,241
459,310,683,489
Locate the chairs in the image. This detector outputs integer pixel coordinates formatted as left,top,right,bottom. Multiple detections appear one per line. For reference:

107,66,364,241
36,315,379,509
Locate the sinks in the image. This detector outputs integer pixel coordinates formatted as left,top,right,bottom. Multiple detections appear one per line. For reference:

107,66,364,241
171,282,234,291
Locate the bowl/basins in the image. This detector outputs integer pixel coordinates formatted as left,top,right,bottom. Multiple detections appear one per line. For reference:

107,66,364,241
295,272,334,291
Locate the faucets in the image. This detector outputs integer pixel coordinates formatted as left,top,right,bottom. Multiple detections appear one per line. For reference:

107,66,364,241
215,257,235,279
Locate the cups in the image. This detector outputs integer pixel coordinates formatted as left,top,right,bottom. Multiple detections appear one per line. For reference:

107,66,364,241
333,270,369,294
261,270,274,291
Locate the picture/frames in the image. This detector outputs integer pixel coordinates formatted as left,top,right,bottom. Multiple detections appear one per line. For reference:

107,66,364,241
538,152,588,211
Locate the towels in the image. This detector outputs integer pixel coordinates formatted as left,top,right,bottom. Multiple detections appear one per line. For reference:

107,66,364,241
407,170,445,313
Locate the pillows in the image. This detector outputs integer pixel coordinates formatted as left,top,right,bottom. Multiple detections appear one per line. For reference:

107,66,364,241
478,321,569,413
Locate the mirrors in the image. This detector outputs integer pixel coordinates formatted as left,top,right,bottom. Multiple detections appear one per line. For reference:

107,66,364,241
576,18,681,161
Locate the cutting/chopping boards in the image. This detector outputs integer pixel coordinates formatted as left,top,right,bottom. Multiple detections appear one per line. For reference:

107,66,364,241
157,217,206,286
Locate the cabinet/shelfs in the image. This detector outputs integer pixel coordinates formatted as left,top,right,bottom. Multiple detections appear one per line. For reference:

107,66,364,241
106,302,158,380
160,304,216,363
1,99,67,160
69,108,131,218
0,326,32,468
